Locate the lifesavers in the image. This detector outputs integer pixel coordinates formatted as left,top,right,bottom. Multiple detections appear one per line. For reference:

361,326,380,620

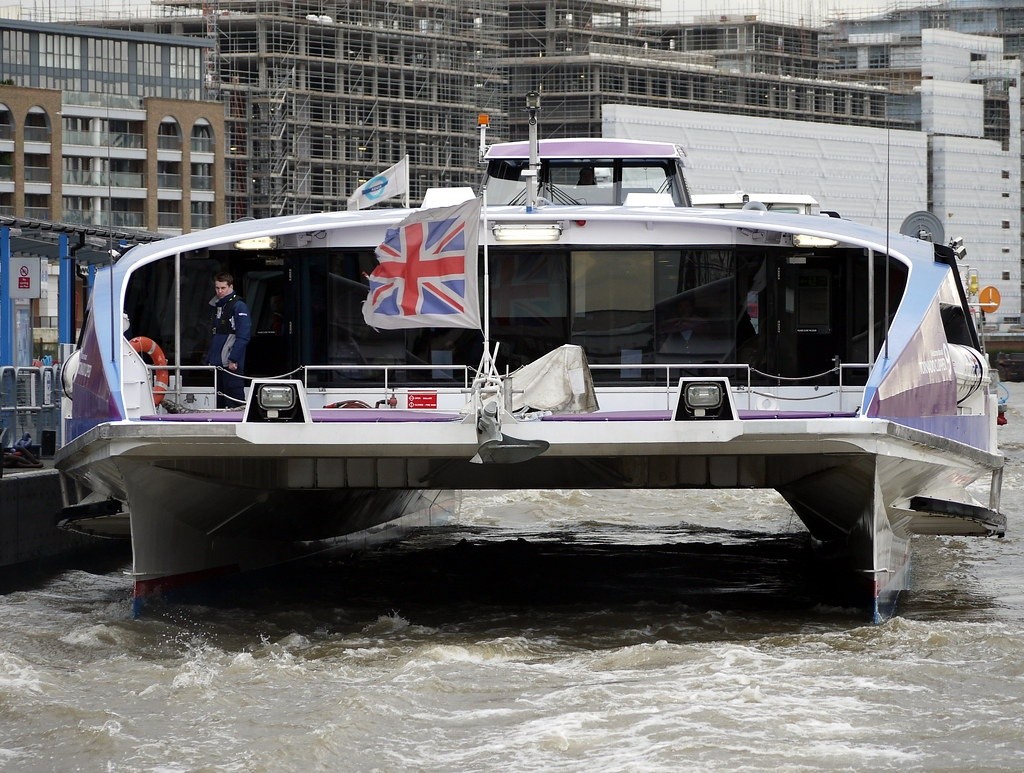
128,335,170,407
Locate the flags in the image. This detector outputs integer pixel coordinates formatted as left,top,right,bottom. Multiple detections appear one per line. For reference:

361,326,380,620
362,194,481,330
347,158,406,211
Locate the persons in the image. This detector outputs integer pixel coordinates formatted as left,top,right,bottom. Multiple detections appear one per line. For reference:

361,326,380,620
207,273,251,409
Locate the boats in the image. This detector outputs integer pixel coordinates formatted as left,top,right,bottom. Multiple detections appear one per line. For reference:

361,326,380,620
50,78,1017,632
